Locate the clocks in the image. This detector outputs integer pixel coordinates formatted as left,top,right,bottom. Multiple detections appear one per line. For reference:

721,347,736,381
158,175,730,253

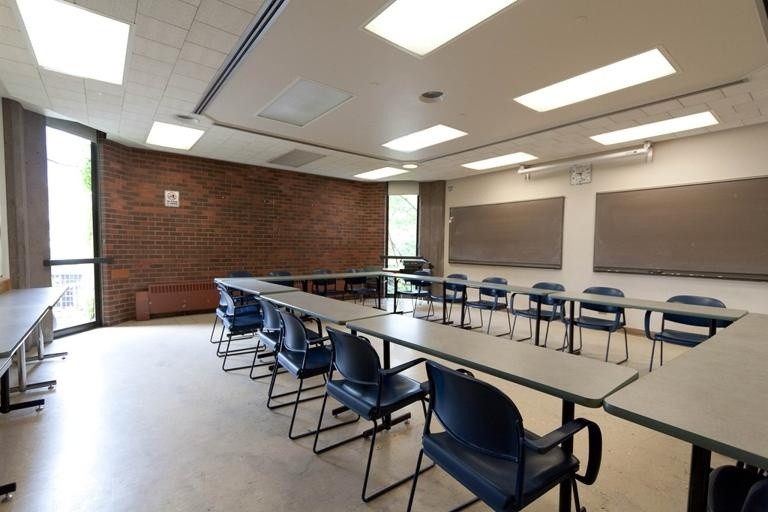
569,161,595,187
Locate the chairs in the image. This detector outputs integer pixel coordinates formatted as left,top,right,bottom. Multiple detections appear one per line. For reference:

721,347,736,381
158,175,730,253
225,269,380,309
558,285,630,367
249,296,329,399
461,275,512,337
310,323,440,504
405,359,605,512
208,279,277,372
642,291,728,375
266,307,363,441
394,271,436,318
426,273,473,326
507,281,570,352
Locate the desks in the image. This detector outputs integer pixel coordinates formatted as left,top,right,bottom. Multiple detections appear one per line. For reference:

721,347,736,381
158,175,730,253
603,311,767,512
0,279,72,507
